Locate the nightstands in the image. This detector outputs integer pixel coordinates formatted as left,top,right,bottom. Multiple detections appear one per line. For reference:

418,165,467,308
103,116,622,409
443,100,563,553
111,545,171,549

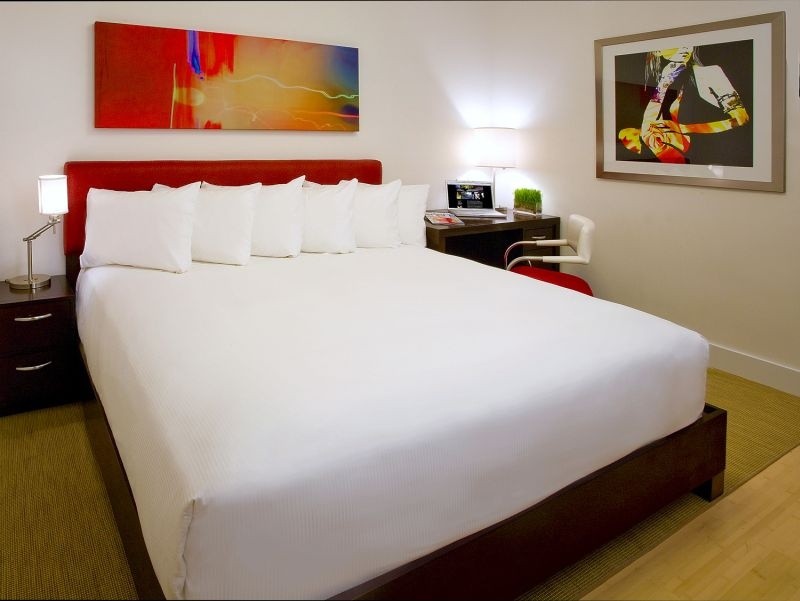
1,276,76,415
425,208,560,276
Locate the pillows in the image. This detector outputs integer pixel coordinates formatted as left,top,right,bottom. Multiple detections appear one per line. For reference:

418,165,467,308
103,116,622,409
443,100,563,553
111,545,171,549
338,180,401,248
398,184,428,248
80,180,203,272
302,178,355,254
251,174,305,258
149,182,263,266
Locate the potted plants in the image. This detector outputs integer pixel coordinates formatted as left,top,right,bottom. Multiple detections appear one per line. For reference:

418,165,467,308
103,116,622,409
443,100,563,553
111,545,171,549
513,188,543,214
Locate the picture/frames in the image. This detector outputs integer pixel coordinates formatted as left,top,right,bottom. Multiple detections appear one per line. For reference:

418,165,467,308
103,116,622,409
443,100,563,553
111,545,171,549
594,11,785,192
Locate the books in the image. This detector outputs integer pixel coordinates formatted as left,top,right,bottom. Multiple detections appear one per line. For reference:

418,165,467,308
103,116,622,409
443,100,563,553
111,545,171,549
424,212,465,226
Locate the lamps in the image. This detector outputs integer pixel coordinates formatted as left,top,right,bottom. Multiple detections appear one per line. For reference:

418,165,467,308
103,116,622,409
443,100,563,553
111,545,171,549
5,174,68,290
476,127,520,210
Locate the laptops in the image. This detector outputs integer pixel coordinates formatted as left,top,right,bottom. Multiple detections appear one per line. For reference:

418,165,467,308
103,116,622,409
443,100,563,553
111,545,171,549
445,181,506,218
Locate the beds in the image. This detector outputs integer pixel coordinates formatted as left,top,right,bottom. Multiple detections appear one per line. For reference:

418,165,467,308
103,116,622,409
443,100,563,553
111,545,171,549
63,160,726,599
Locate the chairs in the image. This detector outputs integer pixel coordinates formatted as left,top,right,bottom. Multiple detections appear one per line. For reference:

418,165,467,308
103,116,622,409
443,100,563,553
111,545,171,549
504,214,594,297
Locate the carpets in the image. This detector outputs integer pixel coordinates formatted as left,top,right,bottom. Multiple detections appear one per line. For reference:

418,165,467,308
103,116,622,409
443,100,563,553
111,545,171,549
1,366,799,600
